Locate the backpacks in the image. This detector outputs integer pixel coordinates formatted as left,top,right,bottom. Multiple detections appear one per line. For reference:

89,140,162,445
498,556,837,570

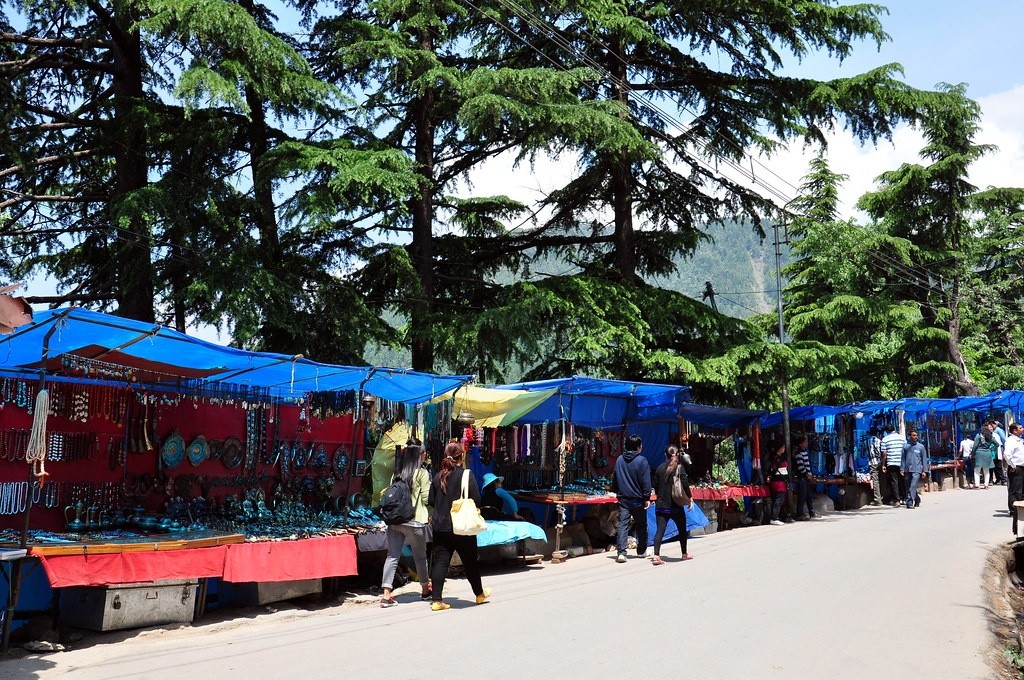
379,467,421,525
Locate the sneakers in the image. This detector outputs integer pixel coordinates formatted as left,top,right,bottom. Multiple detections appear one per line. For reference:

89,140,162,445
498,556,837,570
380,597,398,608
422,590,432,600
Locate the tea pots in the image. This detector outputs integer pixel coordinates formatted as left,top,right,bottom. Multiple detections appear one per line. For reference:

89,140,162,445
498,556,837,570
113,505,133,531
127,505,146,530
139,516,180,533
103,504,117,531
87,501,103,533
64,500,87,534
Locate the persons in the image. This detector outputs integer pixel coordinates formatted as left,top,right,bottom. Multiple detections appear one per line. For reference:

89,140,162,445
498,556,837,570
380,441,431,610
481,471,535,557
867,418,1024,517
653,444,694,565
428,443,490,610
766,437,819,525
163,449,347,534
612,433,652,562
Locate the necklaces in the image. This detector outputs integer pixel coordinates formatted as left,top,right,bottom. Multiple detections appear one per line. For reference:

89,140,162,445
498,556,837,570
361,395,454,442
136,368,360,425
0,377,129,515
460,419,597,488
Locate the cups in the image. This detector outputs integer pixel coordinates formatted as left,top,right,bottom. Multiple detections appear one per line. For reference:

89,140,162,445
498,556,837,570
168,528,180,534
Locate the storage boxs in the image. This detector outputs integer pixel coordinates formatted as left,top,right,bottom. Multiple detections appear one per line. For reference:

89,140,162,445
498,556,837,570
218,578,324,606
56,575,199,632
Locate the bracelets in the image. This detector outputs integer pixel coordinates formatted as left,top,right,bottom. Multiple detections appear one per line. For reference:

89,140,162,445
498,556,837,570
689,499,693,503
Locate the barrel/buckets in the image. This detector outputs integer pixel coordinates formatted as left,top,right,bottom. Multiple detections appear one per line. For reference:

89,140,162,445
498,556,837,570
332,496,345,513
349,492,364,509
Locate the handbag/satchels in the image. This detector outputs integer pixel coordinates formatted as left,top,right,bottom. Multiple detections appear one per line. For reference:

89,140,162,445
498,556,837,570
451,469,487,536
786,478,791,490
672,464,689,506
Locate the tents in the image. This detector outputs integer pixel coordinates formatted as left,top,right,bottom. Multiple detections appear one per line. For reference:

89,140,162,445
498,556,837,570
371,384,560,510
670,389,904,514
894,390,1024,492
487,375,693,552
0,306,477,655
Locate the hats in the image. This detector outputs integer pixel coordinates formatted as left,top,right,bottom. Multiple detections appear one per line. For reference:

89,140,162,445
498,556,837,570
481,473,504,494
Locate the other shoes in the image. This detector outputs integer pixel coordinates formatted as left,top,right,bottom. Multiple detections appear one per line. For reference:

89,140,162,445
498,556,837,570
637,551,650,557
811,513,821,518
519,547,536,556
893,501,900,508
477,588,491,603
914,496,920,506
907,506,915,509
796,515,810,521
682,554,693,560
770,519,785,525
964,480,1008,490
618,555,627,562
653,555,665,565
432,603,450,610
784,517,794,522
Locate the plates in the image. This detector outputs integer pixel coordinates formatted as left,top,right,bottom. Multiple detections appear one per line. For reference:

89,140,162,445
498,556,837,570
209,439,223,461
186,435,210,467
223,437,243,468
162,433,185,468
